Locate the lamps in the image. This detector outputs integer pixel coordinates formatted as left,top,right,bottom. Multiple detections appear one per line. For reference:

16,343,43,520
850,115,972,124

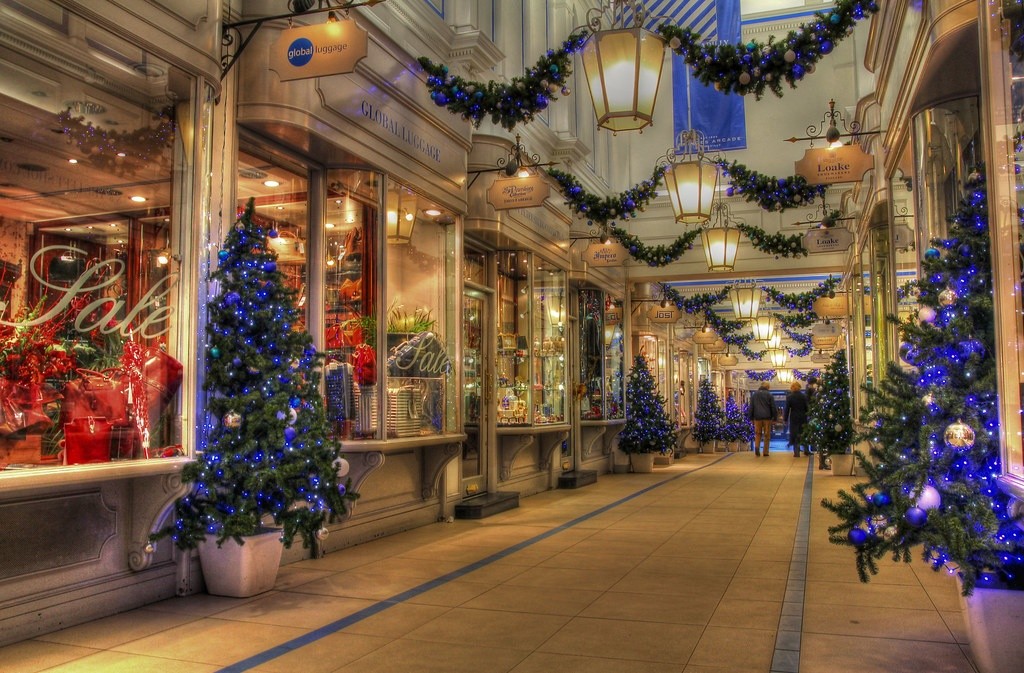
287,0,340,22
695,202,747,273
657,130,719,226
790,195,856,231
579,0,667,136
631,287,675,314
783,97,879,154
770,346,787,369
750,305,776,343
685,316,711,333
725,277,766,322
467,133,560,194
763,327,783,350
569,226,625,250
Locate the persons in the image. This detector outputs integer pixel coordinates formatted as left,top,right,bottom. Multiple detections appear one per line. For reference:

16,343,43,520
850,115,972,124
783,381,815,457
747,381,777,456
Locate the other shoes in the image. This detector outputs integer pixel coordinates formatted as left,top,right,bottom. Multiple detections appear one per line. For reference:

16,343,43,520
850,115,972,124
804,452,813,454
754,447,760,456
763,454,769,456
794,455,799,457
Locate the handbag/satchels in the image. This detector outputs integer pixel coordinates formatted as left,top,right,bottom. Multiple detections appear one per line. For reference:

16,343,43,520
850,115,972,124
339,277,363,301
325,309,378,424
268,231,307,260
60,367,136,465
342,226,365,257
49,250,87,279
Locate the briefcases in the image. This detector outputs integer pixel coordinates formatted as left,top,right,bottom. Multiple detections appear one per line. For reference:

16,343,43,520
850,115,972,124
352,384,423,439
387,332,452,377
